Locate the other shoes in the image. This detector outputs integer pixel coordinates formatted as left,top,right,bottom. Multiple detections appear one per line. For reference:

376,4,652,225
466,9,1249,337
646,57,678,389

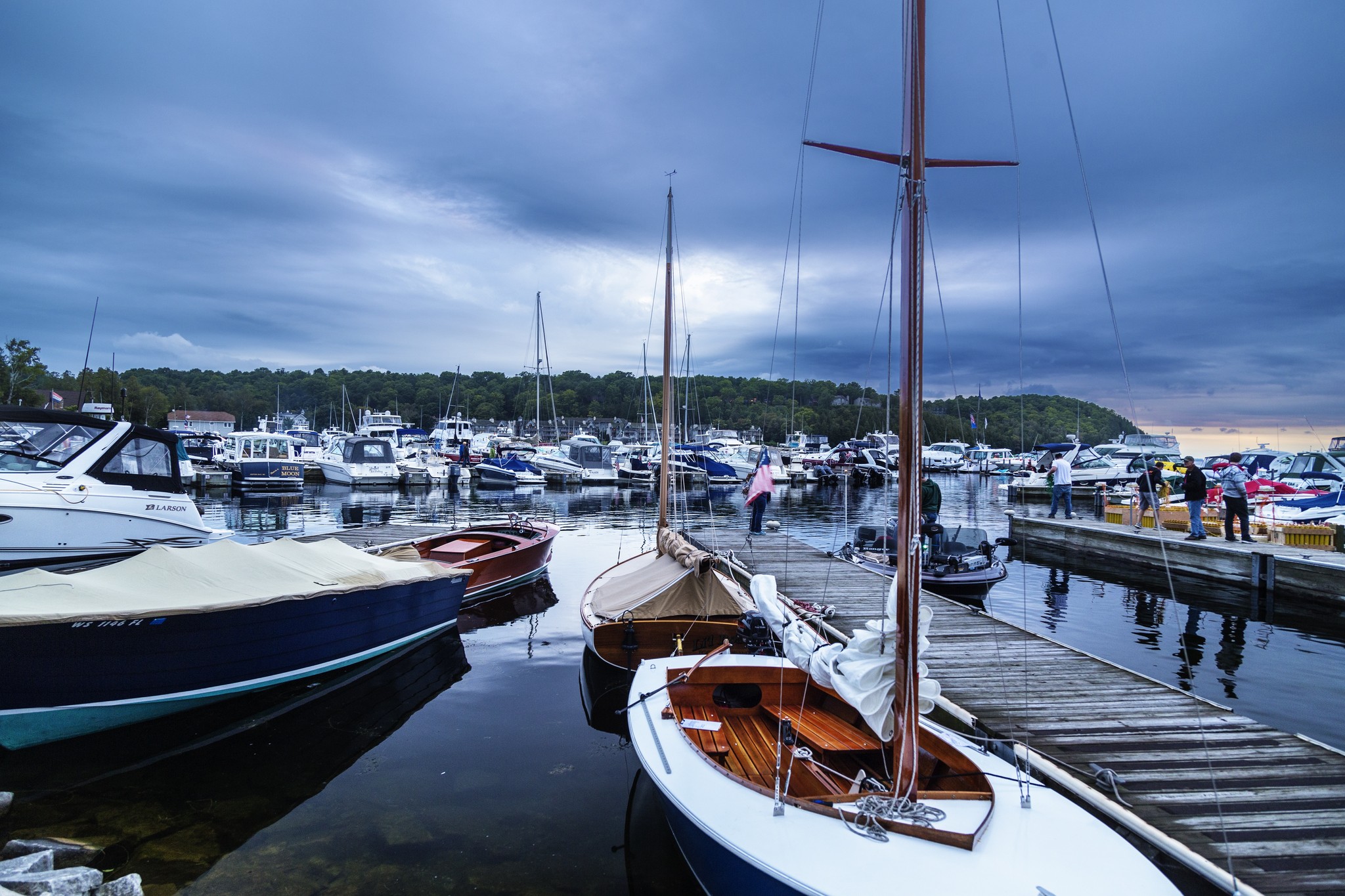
1198,536,1207,540
462,466,466,468
470,465,474,468
1065,517,1072,520
1184,535,1199,540
1048,515,1055,518
465,466,468,468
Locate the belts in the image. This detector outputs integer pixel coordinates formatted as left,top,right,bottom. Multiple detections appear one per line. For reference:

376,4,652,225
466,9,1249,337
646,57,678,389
1058,484,1071,486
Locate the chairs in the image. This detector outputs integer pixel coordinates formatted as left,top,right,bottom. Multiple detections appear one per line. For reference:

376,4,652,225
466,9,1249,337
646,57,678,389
942,541,967,556
871,540,898,554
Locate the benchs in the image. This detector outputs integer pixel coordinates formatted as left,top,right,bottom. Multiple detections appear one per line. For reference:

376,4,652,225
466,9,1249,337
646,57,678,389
674,704,729,768
449,530,530,544
764,702,891,770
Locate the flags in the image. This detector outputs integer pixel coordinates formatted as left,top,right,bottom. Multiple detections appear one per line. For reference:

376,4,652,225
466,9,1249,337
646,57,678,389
51,392,63,403
639,451,641,460
647,462,652,470
641,416,644,423
184,415,190,429
970,410,987,429
724,443,728,454
615,458,619,472
1256,462,1260,476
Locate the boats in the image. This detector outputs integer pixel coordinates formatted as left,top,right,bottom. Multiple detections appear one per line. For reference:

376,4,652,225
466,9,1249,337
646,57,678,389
0,157,1345,566
353,514,560,609
831,517,1017,600
0,527,476,758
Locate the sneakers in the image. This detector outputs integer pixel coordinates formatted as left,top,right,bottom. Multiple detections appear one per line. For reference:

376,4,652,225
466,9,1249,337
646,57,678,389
1134,523,1144,529
750,530,754,534
754,530,766,535
1225,538,1239,542
1241,538,1258,544
1153,525,1167,530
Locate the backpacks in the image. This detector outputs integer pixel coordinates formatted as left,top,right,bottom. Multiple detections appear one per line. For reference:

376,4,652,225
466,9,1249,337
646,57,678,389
741,468,758,498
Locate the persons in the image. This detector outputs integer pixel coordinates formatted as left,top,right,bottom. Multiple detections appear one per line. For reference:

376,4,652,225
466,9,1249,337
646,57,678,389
1134,462,1166,530
456,442,502,468
1178,456,1208,540
1218,452,1258,543
748,458,771,536
921,476,942,552
1026,453,1083,519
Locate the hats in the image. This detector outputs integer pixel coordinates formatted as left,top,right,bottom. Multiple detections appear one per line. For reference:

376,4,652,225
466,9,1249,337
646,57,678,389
1180,456,1194,462
463,442,466,444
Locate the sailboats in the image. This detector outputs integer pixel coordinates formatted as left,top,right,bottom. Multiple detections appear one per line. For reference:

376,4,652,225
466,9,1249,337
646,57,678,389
623,0,1198,896
578,175,793,685
